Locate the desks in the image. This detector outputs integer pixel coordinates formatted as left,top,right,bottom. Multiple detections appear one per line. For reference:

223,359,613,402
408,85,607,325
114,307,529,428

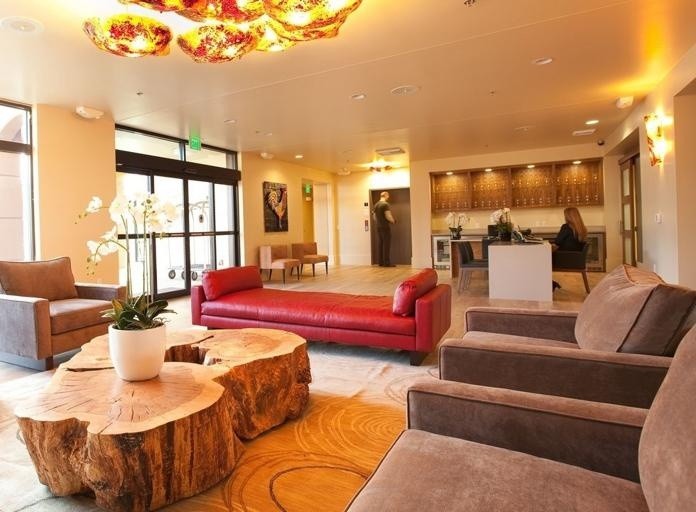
488,240,553,301
14,327,312,512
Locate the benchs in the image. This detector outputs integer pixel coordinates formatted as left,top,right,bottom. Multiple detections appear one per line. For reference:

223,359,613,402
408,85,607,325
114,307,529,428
191,266,451,366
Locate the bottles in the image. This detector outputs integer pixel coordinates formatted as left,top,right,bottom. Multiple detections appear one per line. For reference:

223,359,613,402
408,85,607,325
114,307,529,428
435,174,599,210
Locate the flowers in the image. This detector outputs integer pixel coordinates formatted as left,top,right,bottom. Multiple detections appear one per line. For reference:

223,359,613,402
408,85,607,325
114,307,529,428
74,188,209,329
491,208,512,232
445,212,470,228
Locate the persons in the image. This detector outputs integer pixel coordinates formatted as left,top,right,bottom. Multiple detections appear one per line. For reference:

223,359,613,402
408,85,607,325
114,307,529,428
371,191,397,269
548,205,587,289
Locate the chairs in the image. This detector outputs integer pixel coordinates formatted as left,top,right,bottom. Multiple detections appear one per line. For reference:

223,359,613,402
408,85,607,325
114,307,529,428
456,241,488,293
552,241,590,293
438,264,696,408
0,257,126,372
290,242,328,277
345,322,695,512
260,244,300,284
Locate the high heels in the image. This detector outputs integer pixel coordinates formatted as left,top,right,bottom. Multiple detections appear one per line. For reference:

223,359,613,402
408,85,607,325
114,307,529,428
552,279,561,290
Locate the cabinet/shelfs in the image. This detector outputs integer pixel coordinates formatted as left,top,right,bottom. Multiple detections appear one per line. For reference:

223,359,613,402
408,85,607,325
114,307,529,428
431,236,452,269
585,233,605,273
429,157,604,213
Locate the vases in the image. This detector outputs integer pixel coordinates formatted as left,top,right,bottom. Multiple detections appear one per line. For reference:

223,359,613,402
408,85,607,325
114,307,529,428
498,231,511,242
108,321,166,381
449,227,462,240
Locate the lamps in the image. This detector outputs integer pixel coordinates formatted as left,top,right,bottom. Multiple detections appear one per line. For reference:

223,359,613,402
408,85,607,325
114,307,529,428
82,0,362,64
644,115,663,167
